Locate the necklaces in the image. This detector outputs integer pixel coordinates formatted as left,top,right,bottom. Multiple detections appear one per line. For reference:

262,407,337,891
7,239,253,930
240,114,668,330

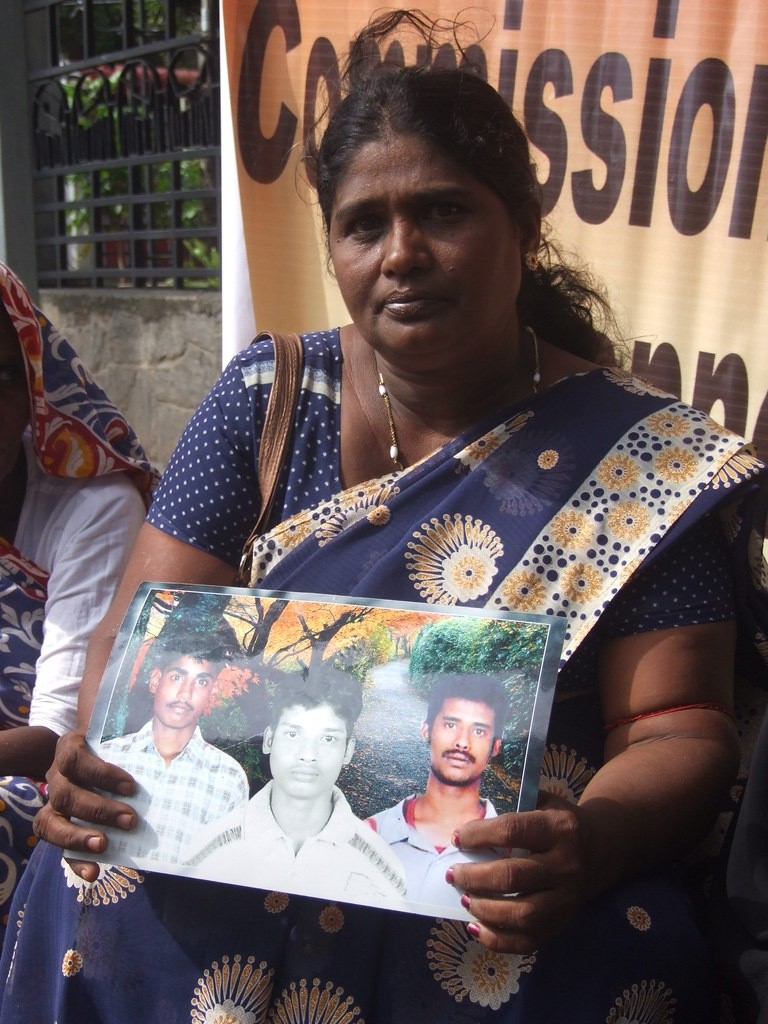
374,318,544,471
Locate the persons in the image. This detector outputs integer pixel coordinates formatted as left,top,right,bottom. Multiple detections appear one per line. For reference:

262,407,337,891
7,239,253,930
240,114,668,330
63,647,508,924
0,66,767,1023
0,262,147,953
